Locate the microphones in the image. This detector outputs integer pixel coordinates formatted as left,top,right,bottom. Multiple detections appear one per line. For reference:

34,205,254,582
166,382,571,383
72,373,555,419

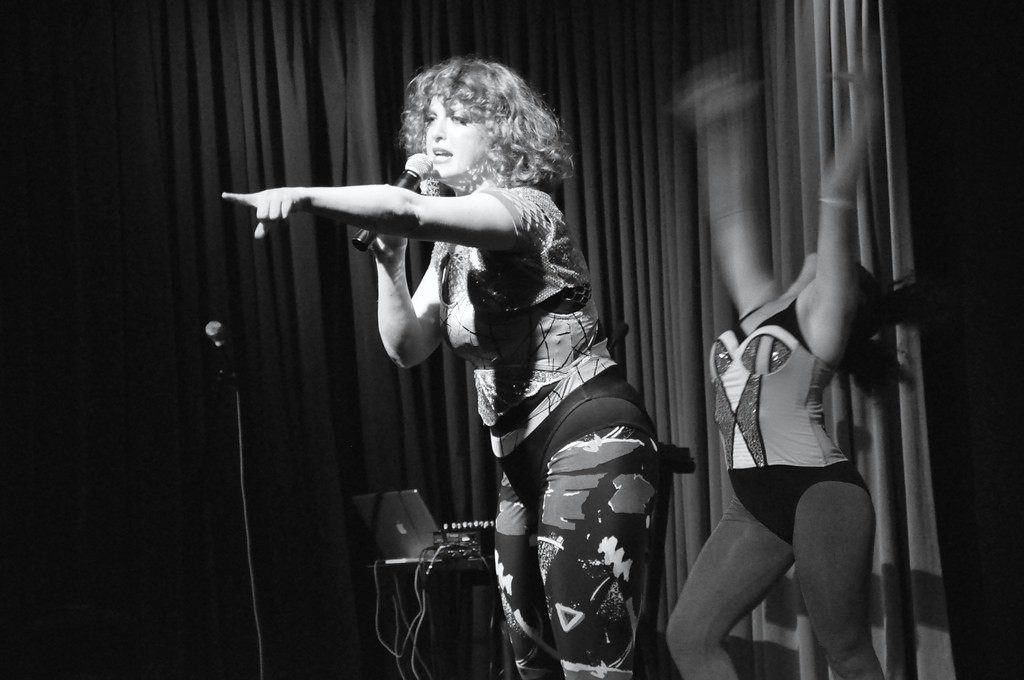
352,153,433,252
205,321,233,375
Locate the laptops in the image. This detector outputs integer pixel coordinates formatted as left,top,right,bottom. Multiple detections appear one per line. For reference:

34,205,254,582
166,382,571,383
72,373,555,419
351,488,444,559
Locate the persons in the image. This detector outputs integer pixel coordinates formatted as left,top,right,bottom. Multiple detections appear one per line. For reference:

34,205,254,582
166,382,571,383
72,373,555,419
222,58,697,680
666,64,924,680
